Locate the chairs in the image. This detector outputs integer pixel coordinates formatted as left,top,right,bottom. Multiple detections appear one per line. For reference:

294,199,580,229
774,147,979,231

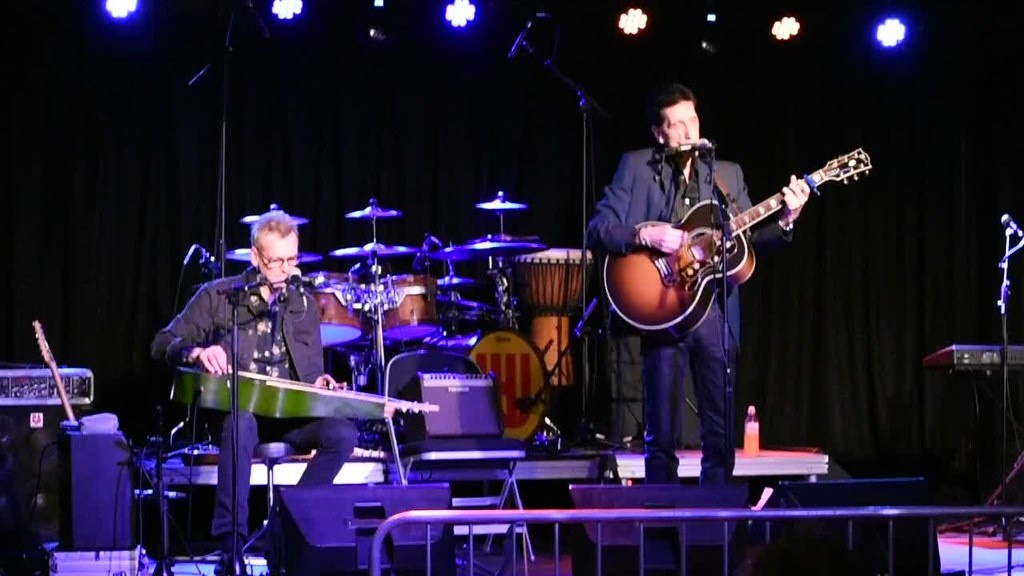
386,348,534,559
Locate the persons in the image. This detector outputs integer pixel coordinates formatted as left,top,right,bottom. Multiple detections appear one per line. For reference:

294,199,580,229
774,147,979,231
151,211,359,575
588,84,809,484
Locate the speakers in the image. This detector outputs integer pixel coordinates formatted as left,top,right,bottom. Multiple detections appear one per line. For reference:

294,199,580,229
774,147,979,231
0,405,135,562
266,482,455,576
400,373,504,441
569,484,749,576
773,478,941,576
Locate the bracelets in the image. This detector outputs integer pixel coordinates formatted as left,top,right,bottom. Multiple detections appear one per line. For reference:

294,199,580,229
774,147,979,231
639,228,651,248
779,220,793,231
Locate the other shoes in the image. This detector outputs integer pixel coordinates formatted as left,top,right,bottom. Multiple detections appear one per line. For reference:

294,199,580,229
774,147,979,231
214,550,247,576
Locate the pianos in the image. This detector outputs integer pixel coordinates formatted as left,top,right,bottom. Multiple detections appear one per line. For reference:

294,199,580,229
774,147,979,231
922,344,1024,531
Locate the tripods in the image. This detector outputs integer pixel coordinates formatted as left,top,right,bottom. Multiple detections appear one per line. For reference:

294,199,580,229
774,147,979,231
532,298,628,452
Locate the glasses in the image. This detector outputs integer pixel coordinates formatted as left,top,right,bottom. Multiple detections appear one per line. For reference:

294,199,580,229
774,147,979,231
257,251,300,269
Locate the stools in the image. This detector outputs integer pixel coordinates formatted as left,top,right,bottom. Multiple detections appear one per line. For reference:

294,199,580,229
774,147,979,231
241,436,312,576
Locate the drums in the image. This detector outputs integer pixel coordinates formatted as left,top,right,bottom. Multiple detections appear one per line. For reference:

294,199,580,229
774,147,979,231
418,327,550,444
367,273,439,343
303,271,367,346
514,246,593,387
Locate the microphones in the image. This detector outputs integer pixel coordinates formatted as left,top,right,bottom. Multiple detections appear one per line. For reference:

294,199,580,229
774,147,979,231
508,17,533,59
247,0,270,39
287,267,305,296
412,237,433,271
200,248,220,274
517,397,536,410
349,259,374,278
696,138,714,151
1000,214,1023,238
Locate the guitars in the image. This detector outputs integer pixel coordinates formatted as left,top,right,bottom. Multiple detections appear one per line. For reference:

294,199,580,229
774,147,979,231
602,146,873,342
33,320,77,421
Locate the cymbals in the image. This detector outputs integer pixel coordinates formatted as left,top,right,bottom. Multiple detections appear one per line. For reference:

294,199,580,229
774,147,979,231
460,234,546,250
241,215,309,227
345,206,401,219
436,295,485,308
475,199,527,210
328,242,419,258
435,275,475,288
223,248,324,264
427,246,472,261
